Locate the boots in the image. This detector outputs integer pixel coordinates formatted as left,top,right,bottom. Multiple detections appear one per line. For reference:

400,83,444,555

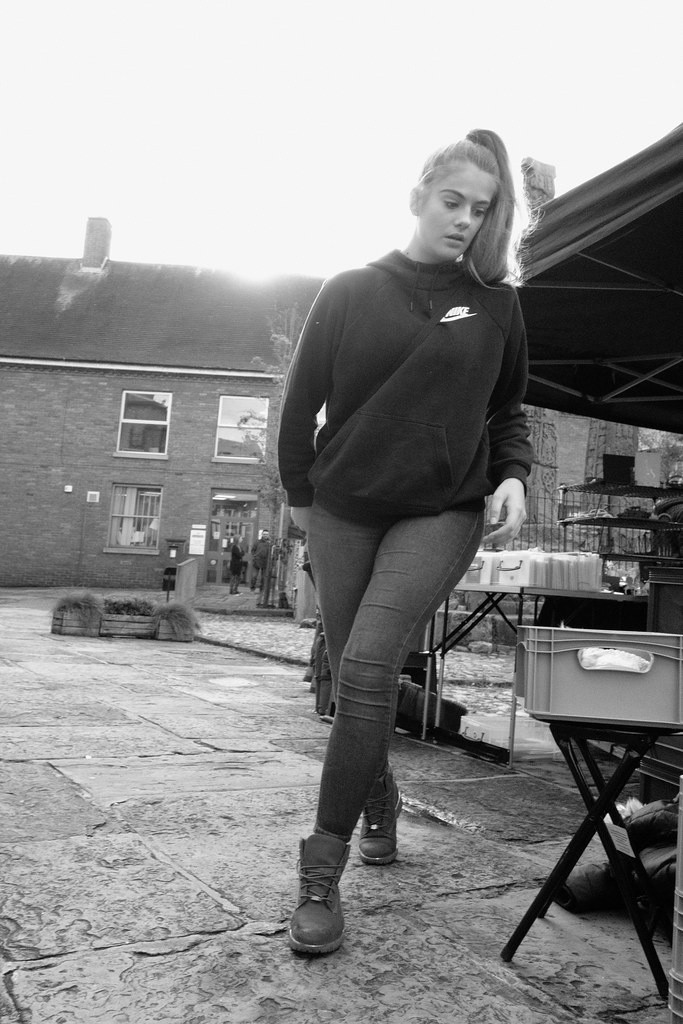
359,766,403,864
290,835,351,952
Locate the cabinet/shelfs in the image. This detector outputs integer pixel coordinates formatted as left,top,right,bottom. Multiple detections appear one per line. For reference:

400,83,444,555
636,566,683,804
560,481,683,563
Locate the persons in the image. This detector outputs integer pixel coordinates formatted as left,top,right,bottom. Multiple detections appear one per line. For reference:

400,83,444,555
230,534,246,595
275,128,537,957
251,529,274,594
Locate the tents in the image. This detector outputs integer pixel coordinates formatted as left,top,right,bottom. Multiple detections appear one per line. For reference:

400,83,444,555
415,121,683,747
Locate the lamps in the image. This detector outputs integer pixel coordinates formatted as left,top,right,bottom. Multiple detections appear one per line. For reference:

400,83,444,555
169,543,178,558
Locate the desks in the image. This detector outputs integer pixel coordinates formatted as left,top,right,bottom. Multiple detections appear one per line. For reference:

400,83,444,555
422,585,646,744
502,718,683,1001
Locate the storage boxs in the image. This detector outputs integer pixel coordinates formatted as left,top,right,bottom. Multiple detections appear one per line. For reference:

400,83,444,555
460,715,560,752
516,625,683,731
667,775,683,1024
